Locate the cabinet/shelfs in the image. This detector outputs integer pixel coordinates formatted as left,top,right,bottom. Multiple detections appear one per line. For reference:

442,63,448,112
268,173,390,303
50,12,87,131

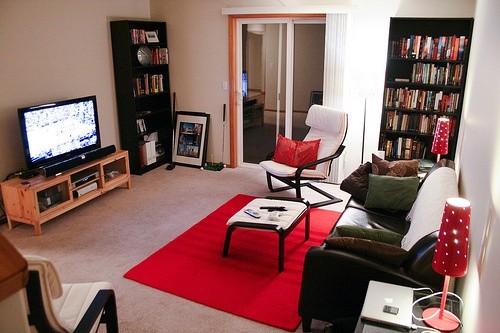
110,21,172,176
0,150,131,236
378,17,474,162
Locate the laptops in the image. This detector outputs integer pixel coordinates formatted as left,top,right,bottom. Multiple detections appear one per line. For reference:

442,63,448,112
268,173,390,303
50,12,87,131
361,281,413,332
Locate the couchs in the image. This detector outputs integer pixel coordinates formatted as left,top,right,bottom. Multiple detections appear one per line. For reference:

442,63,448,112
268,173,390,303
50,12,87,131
297,160,458,333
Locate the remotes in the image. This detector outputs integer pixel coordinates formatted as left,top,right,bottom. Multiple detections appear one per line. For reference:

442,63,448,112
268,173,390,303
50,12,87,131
244,209,261,218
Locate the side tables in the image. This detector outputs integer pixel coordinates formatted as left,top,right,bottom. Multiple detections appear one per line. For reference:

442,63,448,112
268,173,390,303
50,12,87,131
355,292,462,333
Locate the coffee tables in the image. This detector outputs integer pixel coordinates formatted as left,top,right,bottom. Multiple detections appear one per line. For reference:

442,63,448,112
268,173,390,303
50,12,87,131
223,196,311,273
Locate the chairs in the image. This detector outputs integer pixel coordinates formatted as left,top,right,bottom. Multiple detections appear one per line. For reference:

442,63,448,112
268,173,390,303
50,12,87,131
0,231,119,333
259,105,348,208
242,91,265,128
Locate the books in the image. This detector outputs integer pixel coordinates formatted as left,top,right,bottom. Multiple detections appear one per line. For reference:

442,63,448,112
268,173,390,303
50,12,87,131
380,134,426,160
131,29,159,44
385,110,456,137
395,62,464,86
391,35,469,60
151,48,168,64
137,118,146,133
133,74,163,97
384,87,459,113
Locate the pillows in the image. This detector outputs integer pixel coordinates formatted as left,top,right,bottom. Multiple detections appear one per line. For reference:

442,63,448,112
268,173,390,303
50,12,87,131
273,134,321,170
325,153,420,261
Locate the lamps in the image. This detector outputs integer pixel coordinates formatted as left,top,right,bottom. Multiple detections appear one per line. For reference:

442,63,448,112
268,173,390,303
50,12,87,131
421,197,471,332
431,117,450,162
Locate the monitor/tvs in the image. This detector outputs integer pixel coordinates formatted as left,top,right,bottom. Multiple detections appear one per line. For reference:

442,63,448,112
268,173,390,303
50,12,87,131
18,95,101,169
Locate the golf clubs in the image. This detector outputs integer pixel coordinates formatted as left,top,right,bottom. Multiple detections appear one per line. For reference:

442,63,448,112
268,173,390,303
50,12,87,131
166,92,176,170
219,103,228,168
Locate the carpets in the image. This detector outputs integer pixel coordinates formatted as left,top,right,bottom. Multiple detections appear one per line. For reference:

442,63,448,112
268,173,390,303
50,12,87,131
123,194,341,330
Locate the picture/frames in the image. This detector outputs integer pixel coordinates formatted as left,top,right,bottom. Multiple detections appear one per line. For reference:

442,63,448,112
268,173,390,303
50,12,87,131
172,111,210,169
